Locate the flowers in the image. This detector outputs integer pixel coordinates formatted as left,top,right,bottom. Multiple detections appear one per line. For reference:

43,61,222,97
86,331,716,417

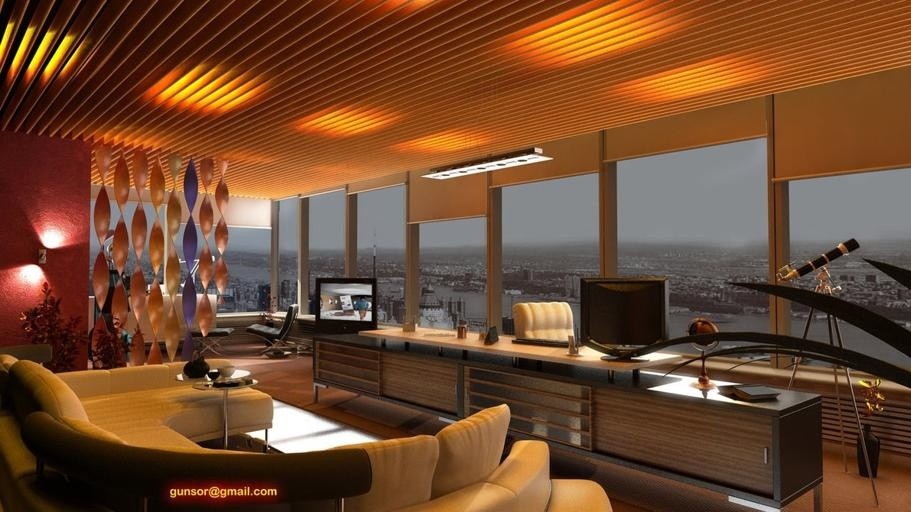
855,379,885,424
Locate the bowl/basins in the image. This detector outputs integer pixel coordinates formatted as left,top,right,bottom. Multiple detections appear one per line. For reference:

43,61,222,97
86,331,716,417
208,371,219,380
217,365,236,378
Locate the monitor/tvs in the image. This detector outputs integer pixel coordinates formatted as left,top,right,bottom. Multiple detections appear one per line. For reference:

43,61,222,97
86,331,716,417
316,278,377,334
581,277,670,362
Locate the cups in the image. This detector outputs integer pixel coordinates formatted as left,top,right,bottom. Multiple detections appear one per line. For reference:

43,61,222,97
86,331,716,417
457,325,467,338
479,328,488,340
404,316,416,332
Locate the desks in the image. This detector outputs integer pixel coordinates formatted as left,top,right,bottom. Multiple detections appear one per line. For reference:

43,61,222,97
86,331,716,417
312,328,823,512
89,293,217,344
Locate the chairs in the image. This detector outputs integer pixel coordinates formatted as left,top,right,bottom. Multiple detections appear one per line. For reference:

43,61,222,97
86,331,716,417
0,344,53,366
190,327,235,358
513,301,575,342
246,304,299,356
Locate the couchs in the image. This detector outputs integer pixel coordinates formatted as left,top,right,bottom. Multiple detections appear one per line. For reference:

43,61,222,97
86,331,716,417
0,354,613,512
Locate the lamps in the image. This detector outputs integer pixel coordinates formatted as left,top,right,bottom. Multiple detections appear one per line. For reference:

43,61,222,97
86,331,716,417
420,147,554,181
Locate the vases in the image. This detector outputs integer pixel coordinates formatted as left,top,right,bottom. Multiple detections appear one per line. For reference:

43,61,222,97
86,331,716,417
857,424,880,479
